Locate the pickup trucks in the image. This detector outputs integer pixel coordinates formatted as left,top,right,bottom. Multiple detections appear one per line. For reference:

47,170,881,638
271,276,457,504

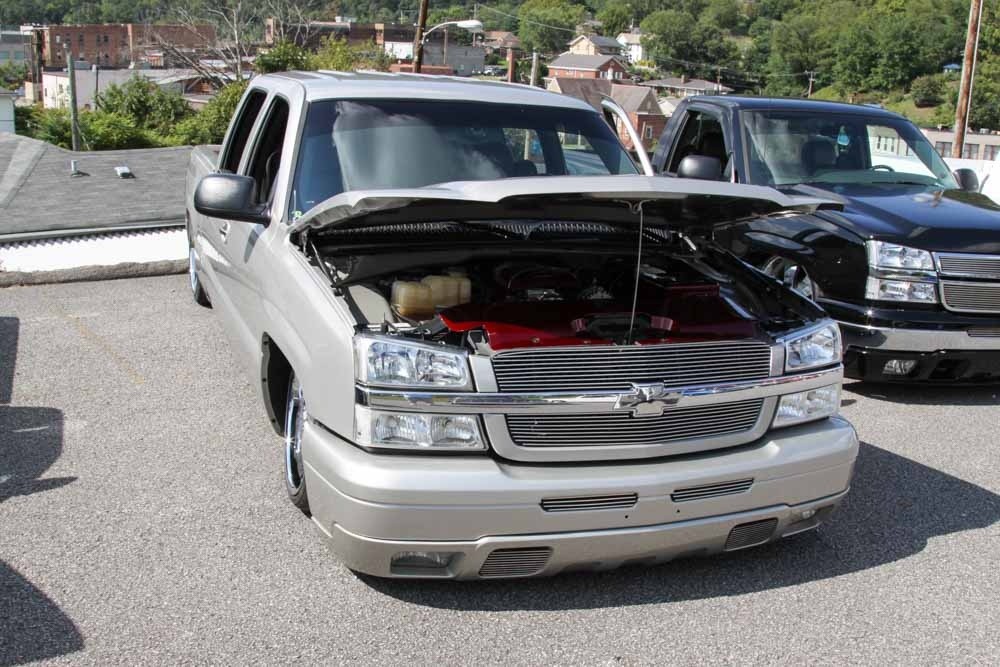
185,70,858,580
563,95,1000,384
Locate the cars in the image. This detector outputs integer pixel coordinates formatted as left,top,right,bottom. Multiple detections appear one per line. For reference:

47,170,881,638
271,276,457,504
483,65,505,77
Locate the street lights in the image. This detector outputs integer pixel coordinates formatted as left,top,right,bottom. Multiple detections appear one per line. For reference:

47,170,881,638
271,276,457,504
412,20,483,73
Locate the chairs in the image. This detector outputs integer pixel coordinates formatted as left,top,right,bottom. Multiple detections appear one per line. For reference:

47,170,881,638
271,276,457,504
266,140,517,197
697,130,841,176
